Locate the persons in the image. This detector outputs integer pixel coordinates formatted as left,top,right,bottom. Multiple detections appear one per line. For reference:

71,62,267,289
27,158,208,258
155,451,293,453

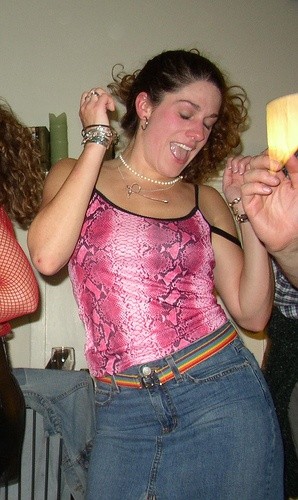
0,94,48,480
262,257,298,500
240,148,298,291
28,49,285,500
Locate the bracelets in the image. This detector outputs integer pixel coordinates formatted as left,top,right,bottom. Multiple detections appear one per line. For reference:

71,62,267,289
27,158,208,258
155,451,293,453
81,124,117,150
229,197,241,210
234,208,249,224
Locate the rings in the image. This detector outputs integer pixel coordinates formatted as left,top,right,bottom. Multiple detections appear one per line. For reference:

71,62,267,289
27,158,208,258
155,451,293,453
91,91,99,97
85,96,90,100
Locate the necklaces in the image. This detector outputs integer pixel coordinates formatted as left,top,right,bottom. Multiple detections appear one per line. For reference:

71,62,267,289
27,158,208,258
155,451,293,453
118,162,176,203
118,152,183,185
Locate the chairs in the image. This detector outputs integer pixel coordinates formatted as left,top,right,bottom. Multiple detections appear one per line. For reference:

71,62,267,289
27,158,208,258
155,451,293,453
0,365,94,500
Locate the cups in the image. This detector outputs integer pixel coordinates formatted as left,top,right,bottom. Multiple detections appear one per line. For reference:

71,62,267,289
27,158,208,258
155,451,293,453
51,347,75,370
